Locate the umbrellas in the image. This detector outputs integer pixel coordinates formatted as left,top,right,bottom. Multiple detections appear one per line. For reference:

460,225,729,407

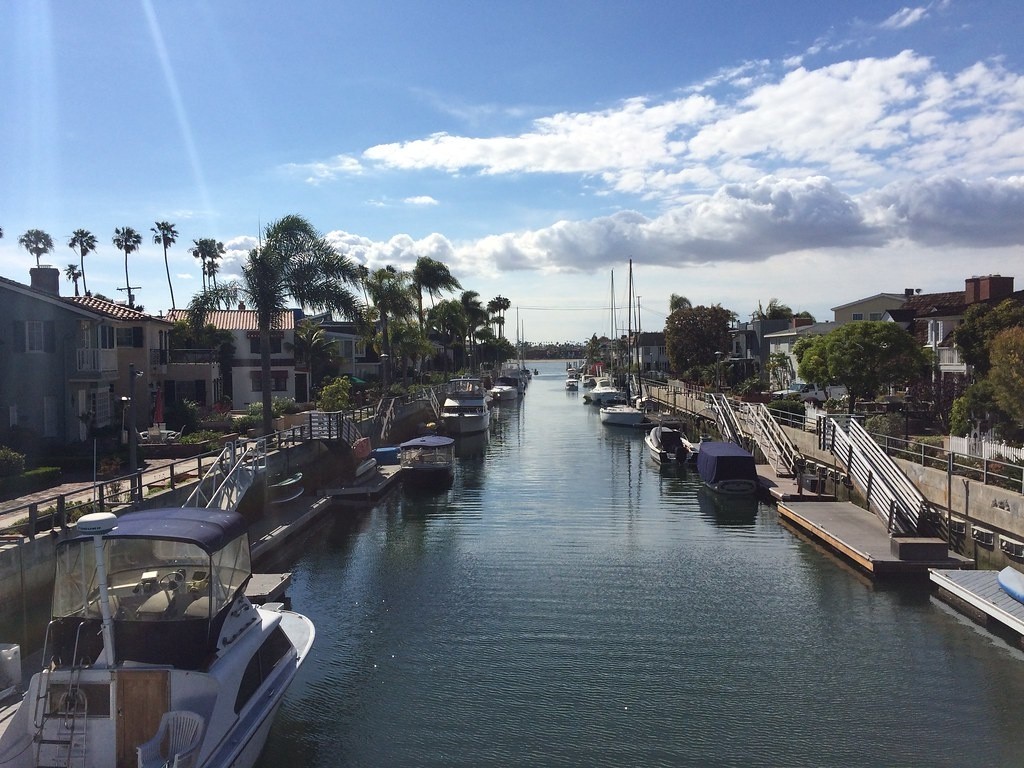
154,388,163,431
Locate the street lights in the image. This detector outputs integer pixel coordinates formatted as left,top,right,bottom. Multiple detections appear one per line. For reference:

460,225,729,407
126,361,145,497
381,353,390,388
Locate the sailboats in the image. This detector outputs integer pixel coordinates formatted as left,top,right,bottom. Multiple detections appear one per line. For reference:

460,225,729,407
490,305,533,406
559,255,655,426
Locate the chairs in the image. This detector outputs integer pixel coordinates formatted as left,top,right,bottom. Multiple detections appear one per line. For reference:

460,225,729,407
167,425,186,443
140,430,177,441
135,709,207,768
152,422,166,430
135,432,148,444
147,427,164,444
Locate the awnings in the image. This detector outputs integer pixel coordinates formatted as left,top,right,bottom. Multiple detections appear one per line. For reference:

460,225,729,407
350,377,367,383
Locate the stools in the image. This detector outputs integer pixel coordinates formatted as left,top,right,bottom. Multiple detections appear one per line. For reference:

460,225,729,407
184,596,217,620
88,595,122,619
136,589,176,621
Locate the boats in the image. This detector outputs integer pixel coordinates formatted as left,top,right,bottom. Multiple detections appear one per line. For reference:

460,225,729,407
7,511,315,768
651,421,696,465
442,375,495,435
398,432,461,490
696,435,769,494
